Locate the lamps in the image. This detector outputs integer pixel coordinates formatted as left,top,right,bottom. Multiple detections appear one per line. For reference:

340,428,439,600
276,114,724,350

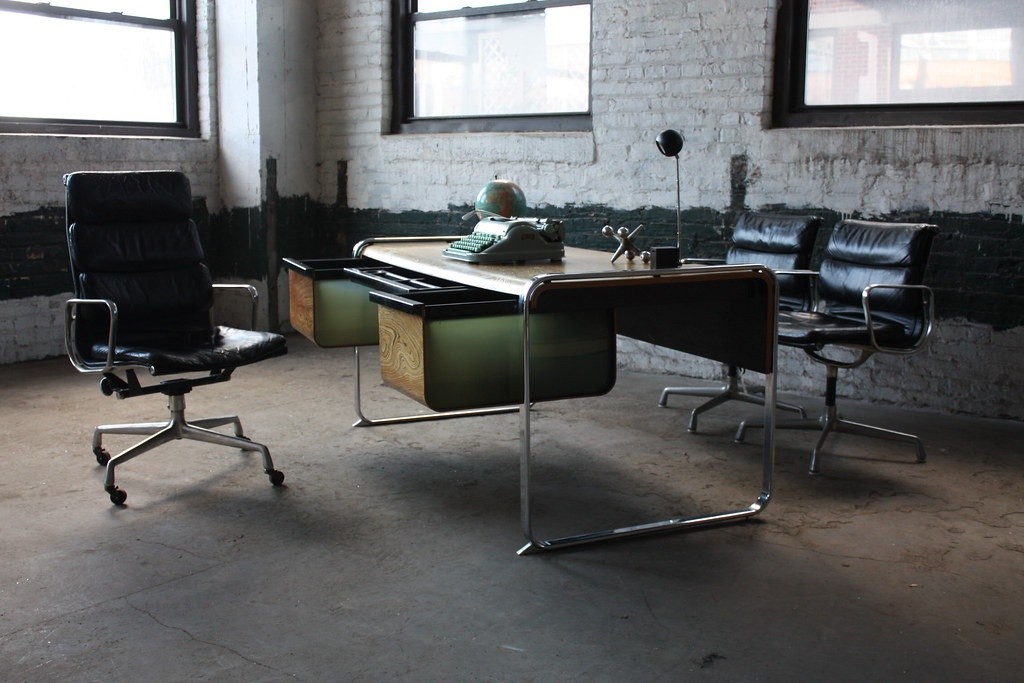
655,130,684,264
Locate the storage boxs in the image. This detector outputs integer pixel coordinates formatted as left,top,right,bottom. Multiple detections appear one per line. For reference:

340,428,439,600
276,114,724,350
650,246,679,275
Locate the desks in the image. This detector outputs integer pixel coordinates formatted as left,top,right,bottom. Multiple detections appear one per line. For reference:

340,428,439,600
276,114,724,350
286,236,775,563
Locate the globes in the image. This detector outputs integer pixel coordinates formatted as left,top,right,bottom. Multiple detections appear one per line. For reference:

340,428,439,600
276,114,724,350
475,174,527,221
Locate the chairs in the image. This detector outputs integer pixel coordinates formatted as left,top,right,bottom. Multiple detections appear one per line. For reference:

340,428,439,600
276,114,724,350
61,167,284,505
659,212,830,444
776,219,939,478
